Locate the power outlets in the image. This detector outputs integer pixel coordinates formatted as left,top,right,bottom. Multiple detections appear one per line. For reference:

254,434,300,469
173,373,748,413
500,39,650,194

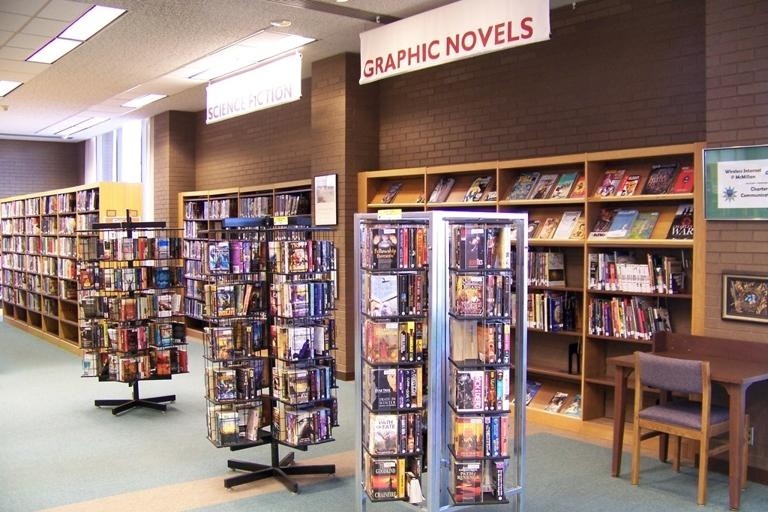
747,427,753,446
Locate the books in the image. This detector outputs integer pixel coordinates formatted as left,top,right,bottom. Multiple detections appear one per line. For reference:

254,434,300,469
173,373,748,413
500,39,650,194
204,357,270,400
79,321,186,352
670,166,694,194
40,216,57,236
270,279,335,318
79,267,186,291
587,208,622,240
566,175,585,198
204,319,270,361
204,314,267,327
640,163,681,195
14,271,27,291
361,313,427,363
549,171,581,198
27,292,42,313
40,195,57,215
447,274,510,318
588,293,673,342
269,318,337,361
509,247,566,287
485,180,497,201
268,239,335,274
84,350,188,383
59,279,77,300
25,217,40,236
665,204,694,240
448,407,510,457
57,258,78,280
537,215,563,239
41,237,57,257
42,277,58,298
363,446,426,504
568,217,585,240
274,194,311,216
361,401,423,454
359,222,429,271
416,193,425,203
207,270,266,284
427,176,455,203
81,292,182,321
271,394,339,445
206,393,272,446
237,225,270,241
207,241,266,274
26,198,40,216
614,174,641,196
562,391,581,419
271,225,311,241
12,201,25,217
2,254,13,269
446,360,511,412
2,236,13,253
209,199,238,219
185,260,210,281
626,210,660,239
12,236,27,255
184,298,208,322
273,272,313,282
510,378,543,406
28,237,40,255
360,270,427,317
14,253,27,272
606,209,639,239
529,173,560,200
186,279,209,300
1,202,13,218
76,214,99,236
239,197,273,219
2,268,13,287
528,215,548,238
270,356,337,405
183,221,207,239
448,453,506,505
543,391,568,413
204,281,268,317
43,297,57,317
78,235,182,261
505,171,541,200
12,218,25,235
2,286,15,305
552,211,582,240
587,249,693,295
76,189,99,212
360,358,423,408
57,194,76,214
510,290,576,333
14,288,28,309
27,274,41,293
183,201,207,219
449,319,512,366
183,241,208,260
58,216,76,237
57,237,78,258
590,168,628,197
462,174,495,201
380,182,405,204
1,219,13,236
41,256,57,278
448,222,512,269
273,318,324,328
27,255,42,274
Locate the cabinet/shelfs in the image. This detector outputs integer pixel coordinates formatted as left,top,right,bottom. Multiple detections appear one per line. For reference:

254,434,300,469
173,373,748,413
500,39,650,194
355,142,706,464
0,183,144,358
354,211,527,512
176,179,311,356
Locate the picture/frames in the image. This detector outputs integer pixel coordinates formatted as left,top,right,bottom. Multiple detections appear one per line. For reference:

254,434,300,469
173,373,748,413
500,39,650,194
720,269,767,325
313,173,337,226
701,144,767,220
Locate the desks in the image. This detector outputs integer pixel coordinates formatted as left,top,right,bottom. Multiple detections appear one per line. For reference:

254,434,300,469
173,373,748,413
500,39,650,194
609,331,766,511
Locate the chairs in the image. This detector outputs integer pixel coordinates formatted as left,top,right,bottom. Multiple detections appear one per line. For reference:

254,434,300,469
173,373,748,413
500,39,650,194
630,351,749,505
